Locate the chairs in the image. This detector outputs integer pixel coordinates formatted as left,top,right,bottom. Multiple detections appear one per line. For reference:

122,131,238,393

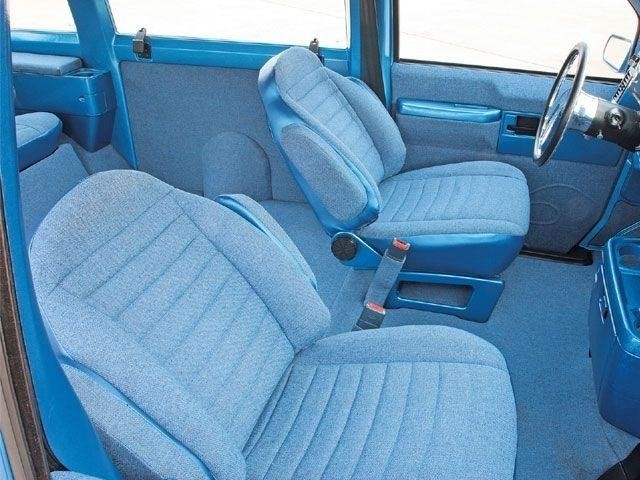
255,45,531,323
16,110,88,244
26,169,519,479
